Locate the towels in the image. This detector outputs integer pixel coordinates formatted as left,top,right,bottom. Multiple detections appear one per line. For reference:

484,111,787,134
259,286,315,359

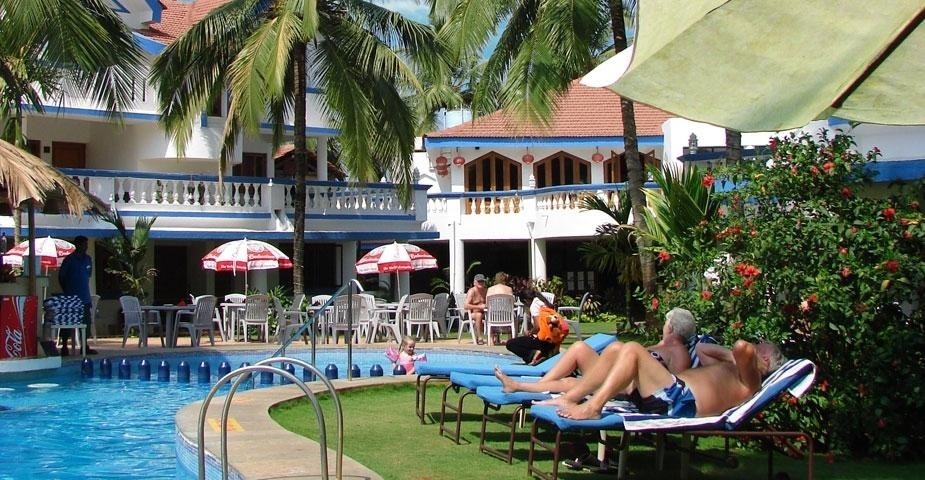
42,293,85,324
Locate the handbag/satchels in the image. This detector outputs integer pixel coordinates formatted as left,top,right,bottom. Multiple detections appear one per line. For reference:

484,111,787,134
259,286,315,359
537,306,569,344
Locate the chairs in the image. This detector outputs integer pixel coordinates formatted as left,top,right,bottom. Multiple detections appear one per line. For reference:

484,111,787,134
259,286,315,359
113,285,592,349
401,334,829,480
89,294,101,344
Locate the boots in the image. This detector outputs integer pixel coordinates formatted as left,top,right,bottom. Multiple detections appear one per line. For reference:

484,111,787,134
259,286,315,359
80,345,98,355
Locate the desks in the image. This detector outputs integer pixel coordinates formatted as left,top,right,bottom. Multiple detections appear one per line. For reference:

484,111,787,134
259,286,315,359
42,322,88,359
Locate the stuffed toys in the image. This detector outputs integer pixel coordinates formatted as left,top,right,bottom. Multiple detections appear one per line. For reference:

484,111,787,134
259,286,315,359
545,314,569,344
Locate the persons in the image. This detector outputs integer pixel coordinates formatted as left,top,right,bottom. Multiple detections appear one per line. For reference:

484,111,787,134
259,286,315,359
384,336,427,375
58,235,98,356
459,274,500,346
531,339,788,422
506,287,560,367
494,307,696,394
485,272,514,346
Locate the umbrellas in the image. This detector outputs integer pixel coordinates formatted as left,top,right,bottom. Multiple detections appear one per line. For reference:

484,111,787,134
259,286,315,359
579,0,925,134
1,235,77,303
355,240,439,303
201,236,292,297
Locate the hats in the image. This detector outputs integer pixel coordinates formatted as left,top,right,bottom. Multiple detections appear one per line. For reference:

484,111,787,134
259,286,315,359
474,274,487,282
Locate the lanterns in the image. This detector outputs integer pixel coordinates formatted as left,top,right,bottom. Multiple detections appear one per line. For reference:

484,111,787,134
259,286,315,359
592,146,604,163
435,154,448,177
522,147,534,164
453,149,465,167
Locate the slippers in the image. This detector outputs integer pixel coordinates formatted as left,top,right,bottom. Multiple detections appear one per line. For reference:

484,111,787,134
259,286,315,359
477,336,484,345
